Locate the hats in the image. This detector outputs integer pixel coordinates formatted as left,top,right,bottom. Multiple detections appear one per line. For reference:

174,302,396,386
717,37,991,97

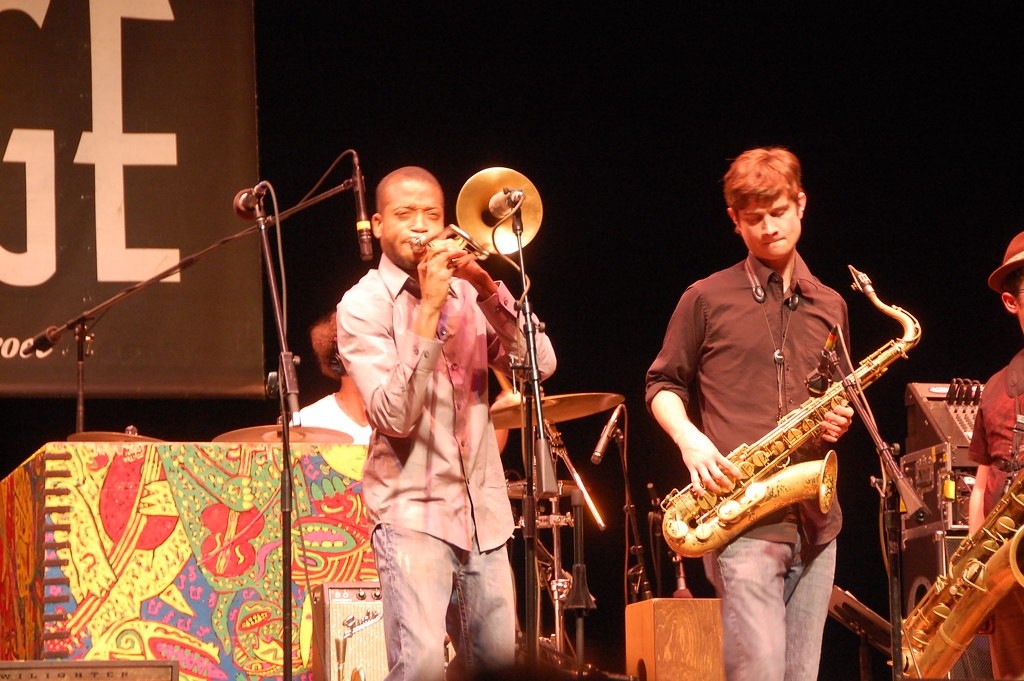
988,231,1024,293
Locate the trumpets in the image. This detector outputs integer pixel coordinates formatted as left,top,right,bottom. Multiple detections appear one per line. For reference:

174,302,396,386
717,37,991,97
411,166,544,267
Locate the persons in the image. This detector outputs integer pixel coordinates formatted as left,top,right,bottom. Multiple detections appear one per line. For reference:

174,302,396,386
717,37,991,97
645,146,854,681
967,231,1024,681
290,314,374,444
334,166,557,681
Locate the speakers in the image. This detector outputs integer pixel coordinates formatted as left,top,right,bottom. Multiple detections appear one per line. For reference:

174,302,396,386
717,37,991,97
311,581,457,681
900,531,995,681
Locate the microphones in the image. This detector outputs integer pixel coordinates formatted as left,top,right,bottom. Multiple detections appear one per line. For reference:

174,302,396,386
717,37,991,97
488,191,524,218
807,325,837,398
591,406,621,464
673,551,692,599
353,154,373,261
233,181,268,219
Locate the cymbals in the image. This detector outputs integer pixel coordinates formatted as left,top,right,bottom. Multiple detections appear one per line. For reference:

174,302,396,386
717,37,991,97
487,392,626,430
65,431,168,443
506,476,591,500
210,424,355,445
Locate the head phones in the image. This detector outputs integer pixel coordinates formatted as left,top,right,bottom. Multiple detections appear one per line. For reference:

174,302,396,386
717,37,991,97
326,335,345,376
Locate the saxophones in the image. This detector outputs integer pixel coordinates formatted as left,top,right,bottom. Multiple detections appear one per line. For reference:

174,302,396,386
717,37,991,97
658,264,924,558
885,475,1024,681
